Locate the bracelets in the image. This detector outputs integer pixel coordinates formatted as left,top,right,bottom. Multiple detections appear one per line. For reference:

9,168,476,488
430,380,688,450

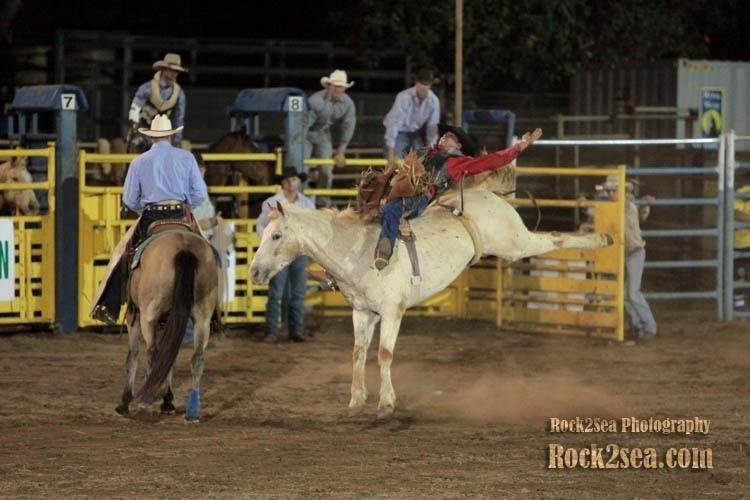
521,139,529,144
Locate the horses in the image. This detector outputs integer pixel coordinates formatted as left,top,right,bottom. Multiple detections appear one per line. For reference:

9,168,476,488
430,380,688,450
247,144,614,421
86,124,274,220
114,223,219,425
0,145,41,216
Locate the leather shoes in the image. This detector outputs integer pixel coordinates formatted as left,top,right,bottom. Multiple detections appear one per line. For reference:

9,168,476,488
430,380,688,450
376,238,391,269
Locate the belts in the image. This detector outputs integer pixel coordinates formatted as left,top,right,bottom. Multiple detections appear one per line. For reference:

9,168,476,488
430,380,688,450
145,204,182,213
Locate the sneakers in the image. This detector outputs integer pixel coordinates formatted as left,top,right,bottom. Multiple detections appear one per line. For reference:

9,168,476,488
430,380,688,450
624,328,655,344
260,333,305,342
93,310,116,327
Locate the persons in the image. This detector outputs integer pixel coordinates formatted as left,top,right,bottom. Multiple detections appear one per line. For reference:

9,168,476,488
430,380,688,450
383,66,441,162
307,70,357,208
120,52,188,217
255,166,317,342
374,125,543,271
93,116,223,331
578,164,659,341
733,166,750,317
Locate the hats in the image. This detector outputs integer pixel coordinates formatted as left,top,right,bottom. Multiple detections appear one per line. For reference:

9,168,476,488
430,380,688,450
321,70,354,88
438,123,480,157
138,114,183,137
411,68,443,87
275,166,308,184
595,175,635,191
152,54,188,73
